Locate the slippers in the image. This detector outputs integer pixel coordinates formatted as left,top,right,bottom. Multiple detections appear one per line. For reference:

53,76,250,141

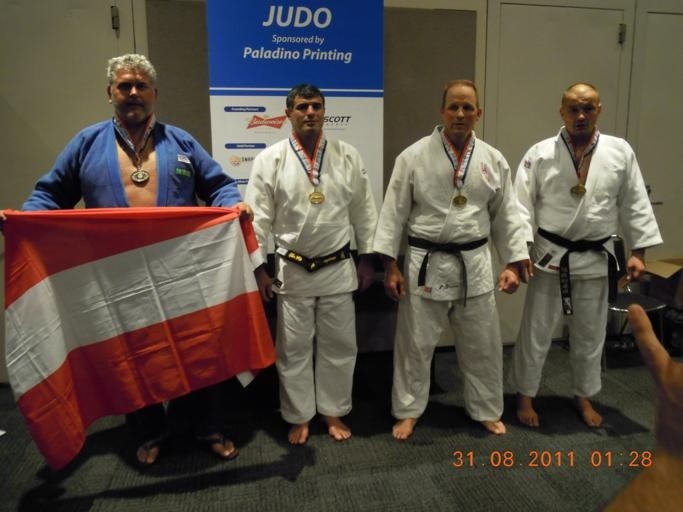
198,434,237,460
136,433,167,464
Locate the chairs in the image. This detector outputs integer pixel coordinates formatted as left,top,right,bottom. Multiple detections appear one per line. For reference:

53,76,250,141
563,234,667,359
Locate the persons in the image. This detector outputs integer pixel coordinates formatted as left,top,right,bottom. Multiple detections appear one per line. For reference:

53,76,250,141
241,84,377,444
0,54,256,466
513,83,665,427
605,303,683,510
375,80,530,441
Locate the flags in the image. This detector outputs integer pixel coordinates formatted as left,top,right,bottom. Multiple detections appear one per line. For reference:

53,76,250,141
1,206,277,471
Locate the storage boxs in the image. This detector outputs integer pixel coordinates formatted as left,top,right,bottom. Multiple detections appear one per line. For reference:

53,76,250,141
644,256,683,309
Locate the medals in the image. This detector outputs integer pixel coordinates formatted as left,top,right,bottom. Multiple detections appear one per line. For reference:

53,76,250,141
132,168,150,183
451,195,468,208
571,183,586,198
308,191,325,205
